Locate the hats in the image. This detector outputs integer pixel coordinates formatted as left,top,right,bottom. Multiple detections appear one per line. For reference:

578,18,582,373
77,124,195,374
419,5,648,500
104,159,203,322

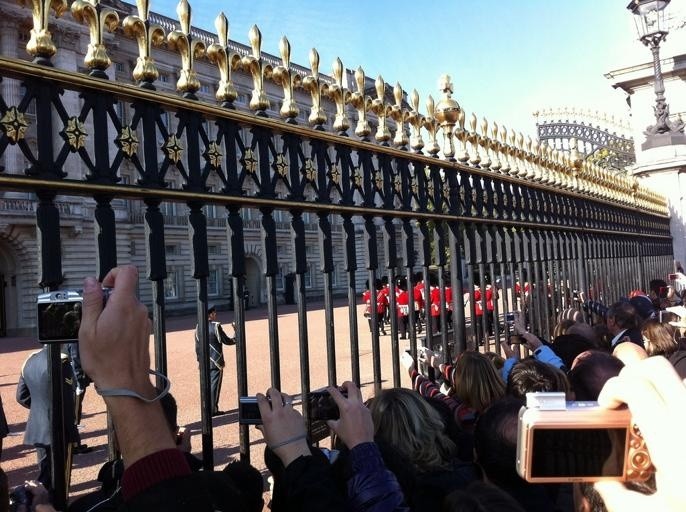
207,303,216,314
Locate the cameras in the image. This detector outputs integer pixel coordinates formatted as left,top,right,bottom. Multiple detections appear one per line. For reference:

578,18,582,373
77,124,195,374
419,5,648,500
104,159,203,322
668,274,679,281
405,350,420,360
660,311,679,323
315,391,348,421
516,402,654,484
239,397,284,424
36,287,109,343
9,483,29,506
573,290,578,300
505,314,519,322
660,287,668,298
506,334,527,345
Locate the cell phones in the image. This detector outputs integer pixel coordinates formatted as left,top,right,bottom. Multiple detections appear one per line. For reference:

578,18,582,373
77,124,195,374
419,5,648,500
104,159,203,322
176,427,186,446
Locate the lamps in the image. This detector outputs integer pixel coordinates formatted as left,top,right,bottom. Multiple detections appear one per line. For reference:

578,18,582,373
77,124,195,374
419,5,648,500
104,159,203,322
626,0,686,151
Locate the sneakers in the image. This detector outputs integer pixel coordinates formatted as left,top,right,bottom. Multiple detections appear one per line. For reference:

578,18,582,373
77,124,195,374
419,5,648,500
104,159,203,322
214,411,225,416
73,444,92,454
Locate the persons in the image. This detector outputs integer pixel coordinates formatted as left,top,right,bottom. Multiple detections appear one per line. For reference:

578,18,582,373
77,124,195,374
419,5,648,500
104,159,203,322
0,259,685,509
